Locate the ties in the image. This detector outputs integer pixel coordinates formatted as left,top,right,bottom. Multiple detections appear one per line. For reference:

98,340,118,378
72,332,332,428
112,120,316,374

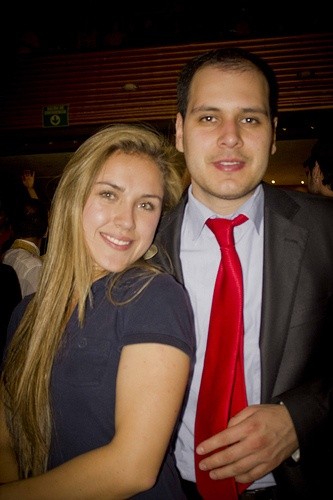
193,213,256,500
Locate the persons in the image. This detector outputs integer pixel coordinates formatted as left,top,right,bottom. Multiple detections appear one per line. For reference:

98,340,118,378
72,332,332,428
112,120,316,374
140,47,333,500
0,124,197,500
303,131,333,200
0,168,54,334
